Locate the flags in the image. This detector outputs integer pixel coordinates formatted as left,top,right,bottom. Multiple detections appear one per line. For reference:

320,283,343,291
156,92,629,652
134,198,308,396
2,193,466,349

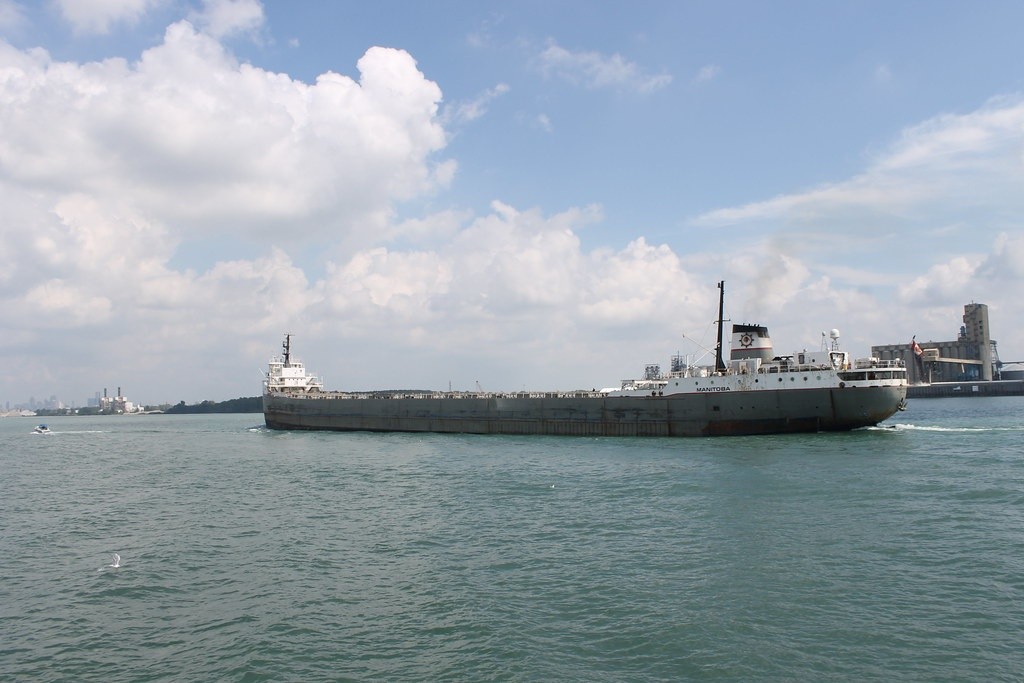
911,341,925,359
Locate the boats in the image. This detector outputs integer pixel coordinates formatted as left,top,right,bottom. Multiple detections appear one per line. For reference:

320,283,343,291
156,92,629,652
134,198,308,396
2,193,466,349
262,280,906,436
34,423,50,434
